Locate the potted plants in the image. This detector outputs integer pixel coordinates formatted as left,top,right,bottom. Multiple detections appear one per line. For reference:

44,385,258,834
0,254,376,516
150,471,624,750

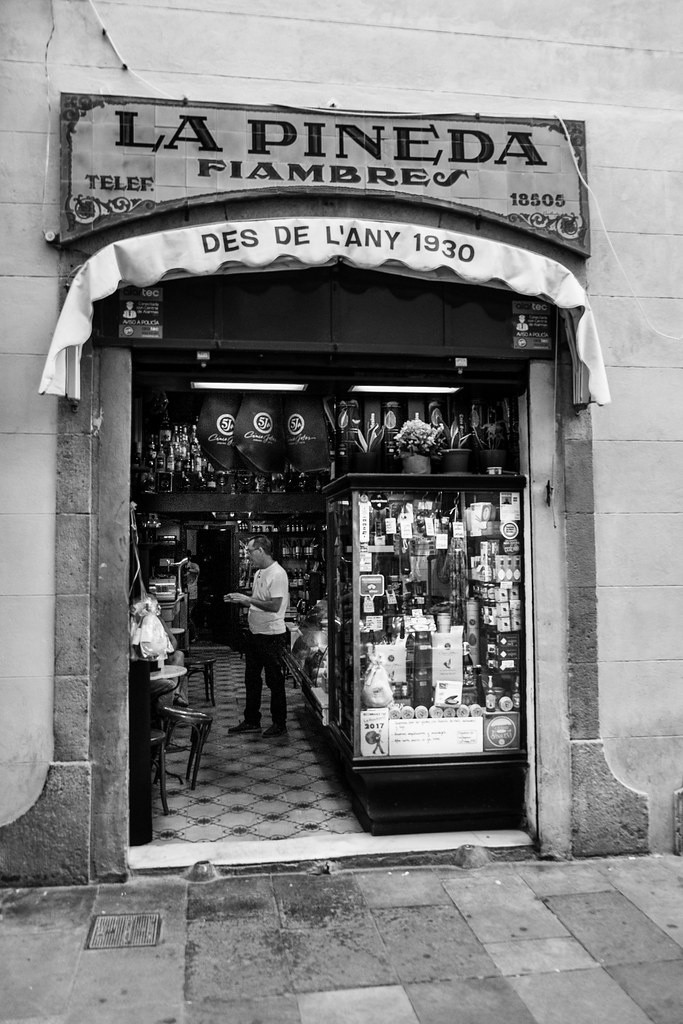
348,403,513,475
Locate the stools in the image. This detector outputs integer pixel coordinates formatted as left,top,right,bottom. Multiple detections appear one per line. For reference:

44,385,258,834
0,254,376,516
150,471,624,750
151,651,217,816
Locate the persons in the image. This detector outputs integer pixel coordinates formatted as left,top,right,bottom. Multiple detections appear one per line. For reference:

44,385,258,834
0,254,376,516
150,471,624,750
223,535,288,738
187,549,200,641
130,593,189,717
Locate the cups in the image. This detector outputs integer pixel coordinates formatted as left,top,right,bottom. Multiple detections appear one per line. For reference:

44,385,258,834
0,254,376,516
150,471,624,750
437,613,452,633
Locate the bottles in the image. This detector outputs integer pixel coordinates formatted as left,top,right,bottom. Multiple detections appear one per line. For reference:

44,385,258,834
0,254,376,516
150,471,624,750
298,591,310,614
476,665,485,707
133,415,217,492
282,538,314,556
391,683,397,700
512,677,520,711
284,567,303,589
464,642,474,687
401,684,408,699
285,519,305,532
485,676,496,711
